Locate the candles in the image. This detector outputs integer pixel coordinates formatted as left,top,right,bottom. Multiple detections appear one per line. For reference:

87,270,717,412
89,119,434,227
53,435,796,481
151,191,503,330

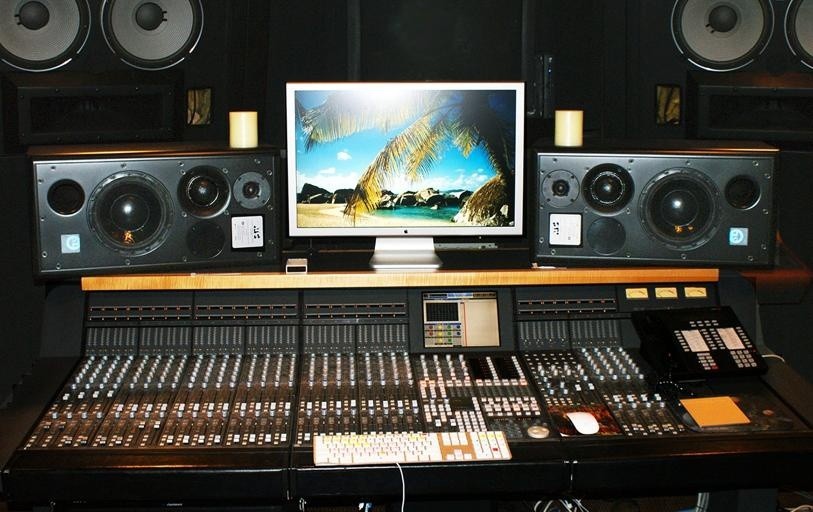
227,110,260,149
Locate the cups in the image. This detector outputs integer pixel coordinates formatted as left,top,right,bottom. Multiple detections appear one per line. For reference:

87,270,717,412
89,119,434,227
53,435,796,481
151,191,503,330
228,111,258,148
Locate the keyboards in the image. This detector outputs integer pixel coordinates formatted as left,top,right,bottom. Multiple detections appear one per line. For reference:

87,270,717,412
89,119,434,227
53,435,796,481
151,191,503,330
313,430,513,467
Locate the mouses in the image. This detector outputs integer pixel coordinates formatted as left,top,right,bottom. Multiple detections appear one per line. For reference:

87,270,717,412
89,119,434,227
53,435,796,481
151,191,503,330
565,410,600,434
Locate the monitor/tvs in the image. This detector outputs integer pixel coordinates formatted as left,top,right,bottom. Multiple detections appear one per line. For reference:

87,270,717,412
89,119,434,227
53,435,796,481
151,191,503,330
287,81,524,271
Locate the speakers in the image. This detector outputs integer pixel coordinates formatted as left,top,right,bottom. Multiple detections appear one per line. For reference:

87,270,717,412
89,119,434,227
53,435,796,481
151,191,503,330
0,0,204,75
670,0,813,76
534,140,780,269
29,142,284,288
